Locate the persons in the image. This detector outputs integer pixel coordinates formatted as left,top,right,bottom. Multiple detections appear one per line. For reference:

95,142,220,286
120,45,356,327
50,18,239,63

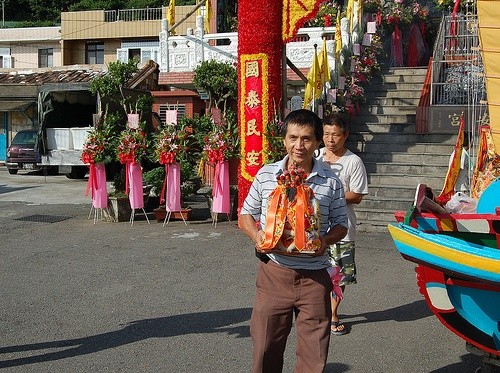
448,131,475,197
239,108,349,373
313,112,369,336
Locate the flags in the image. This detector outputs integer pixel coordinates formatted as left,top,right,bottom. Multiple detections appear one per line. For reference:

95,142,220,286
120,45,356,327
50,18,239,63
303,0,363,108
436,115,464,202
166,0,213,36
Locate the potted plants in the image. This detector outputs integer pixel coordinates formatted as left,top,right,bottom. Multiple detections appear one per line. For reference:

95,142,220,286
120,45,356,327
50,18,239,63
108,168,132,223
143,164,201,221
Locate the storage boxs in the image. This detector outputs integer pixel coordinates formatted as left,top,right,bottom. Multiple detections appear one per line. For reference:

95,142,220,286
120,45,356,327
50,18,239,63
45,128,74,150
70,127,94,151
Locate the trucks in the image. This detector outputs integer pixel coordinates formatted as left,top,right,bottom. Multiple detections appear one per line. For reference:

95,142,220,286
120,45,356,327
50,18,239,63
34,81,122,179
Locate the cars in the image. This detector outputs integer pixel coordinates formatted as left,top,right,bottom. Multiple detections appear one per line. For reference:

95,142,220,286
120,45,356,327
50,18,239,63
4,129,57,175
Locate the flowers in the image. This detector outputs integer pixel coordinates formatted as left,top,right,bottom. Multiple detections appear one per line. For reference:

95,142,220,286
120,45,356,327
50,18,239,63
316,0,429,117
81,125,238,164
264,122,288,163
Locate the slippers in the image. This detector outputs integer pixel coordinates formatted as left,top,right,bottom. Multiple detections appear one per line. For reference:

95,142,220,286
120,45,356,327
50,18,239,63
331,321,347,335
